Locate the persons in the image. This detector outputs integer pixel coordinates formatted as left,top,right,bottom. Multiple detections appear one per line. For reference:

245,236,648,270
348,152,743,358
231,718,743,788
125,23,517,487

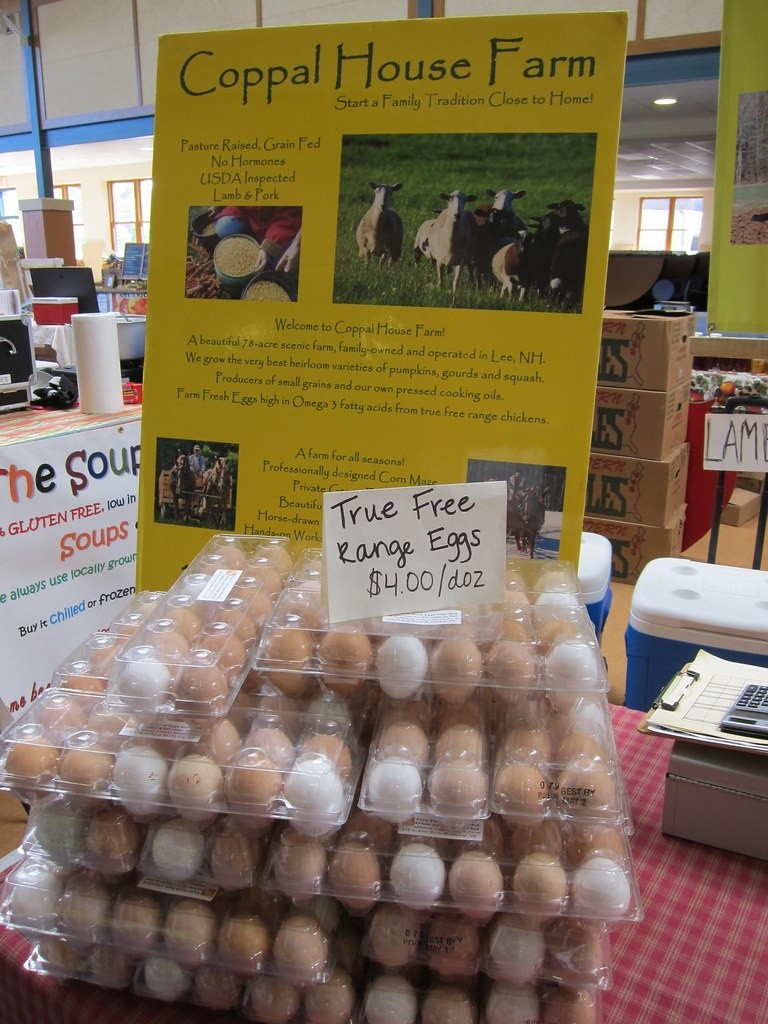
507,469,527,501
208,206,304,274
189,445,205,471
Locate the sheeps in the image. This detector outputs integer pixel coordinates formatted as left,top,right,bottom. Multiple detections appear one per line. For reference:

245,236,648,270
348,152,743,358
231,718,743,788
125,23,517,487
352,178,406,268
408,189,593,306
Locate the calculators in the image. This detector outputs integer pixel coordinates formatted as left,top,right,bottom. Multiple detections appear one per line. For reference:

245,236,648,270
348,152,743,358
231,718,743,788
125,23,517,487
719,683,768,738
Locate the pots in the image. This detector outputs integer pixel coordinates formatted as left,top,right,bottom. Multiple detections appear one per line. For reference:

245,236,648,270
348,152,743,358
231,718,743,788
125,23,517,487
116,314,146,361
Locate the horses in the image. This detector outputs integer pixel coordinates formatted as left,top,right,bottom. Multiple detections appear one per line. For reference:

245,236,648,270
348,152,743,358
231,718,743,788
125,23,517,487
506,483,549,556
170,454,230,530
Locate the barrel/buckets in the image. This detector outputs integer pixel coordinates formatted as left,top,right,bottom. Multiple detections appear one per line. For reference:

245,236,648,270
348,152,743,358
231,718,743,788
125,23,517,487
191,211,298,302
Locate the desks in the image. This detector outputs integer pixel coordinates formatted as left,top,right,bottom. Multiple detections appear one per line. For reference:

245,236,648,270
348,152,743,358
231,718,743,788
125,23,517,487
0,704,768,1024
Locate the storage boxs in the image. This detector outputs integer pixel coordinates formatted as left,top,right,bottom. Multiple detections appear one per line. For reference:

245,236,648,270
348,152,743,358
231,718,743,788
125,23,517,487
584,442,689,527
583,504,688,586
597,310,694,390
589,387,689,460
661,740,768,860
622,558,768,714
32,296,77,325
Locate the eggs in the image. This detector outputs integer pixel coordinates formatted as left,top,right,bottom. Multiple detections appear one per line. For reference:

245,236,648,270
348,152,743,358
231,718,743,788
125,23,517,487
0,541,631,1024
